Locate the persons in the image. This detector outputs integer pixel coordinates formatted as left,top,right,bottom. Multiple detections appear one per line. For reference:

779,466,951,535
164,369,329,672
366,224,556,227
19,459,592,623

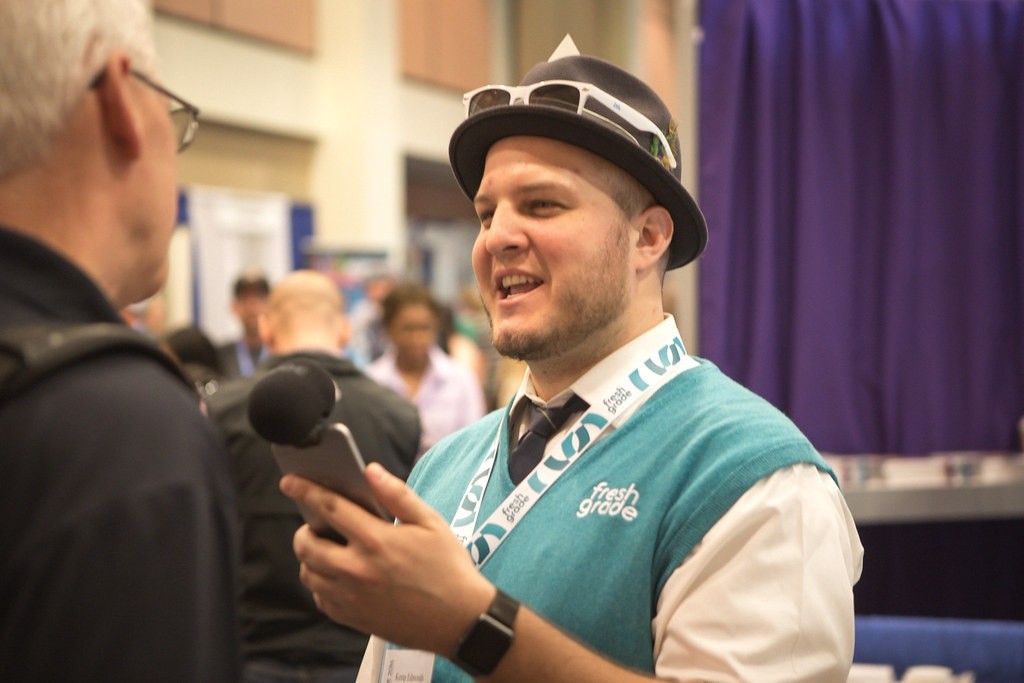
200,270,421,683
362,286,486,455
279,55,864,683
219,273,273,379
158,328,222,394
0,0,241,683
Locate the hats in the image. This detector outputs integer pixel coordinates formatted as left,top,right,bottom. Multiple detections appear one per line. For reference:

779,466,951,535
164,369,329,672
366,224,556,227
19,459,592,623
448,55,710,271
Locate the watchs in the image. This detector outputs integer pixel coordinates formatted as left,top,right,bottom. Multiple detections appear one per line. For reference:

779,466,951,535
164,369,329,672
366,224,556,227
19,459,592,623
450,590,519,676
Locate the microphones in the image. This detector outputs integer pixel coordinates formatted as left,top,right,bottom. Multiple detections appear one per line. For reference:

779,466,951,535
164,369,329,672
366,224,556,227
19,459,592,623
248,359,336,448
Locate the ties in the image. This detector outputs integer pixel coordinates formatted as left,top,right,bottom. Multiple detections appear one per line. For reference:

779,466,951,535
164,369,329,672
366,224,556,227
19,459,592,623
505,393,590,487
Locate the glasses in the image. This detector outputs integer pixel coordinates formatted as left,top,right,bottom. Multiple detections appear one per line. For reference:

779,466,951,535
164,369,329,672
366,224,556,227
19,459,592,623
88,60,202,154
462,79,678,169
